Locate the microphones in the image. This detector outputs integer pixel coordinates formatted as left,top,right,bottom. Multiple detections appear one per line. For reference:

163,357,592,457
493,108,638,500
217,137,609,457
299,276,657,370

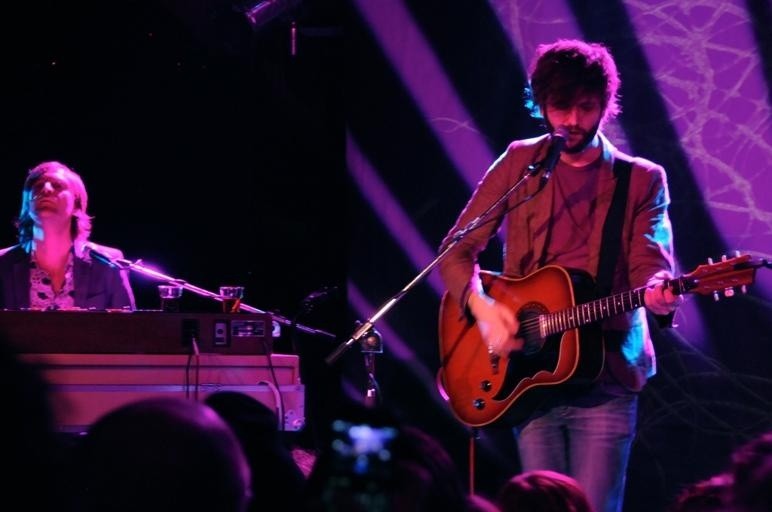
81,244,121,272
537,128,570,191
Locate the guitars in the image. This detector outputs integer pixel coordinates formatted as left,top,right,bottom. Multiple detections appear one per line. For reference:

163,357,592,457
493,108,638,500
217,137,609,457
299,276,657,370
439,251,756,429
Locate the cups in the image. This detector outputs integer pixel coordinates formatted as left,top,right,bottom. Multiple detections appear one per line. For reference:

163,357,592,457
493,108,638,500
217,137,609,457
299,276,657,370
158,285,184,311
220,287,244,313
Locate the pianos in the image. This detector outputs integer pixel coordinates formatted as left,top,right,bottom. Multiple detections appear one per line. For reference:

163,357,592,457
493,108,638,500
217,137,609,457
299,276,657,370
18,354,304,430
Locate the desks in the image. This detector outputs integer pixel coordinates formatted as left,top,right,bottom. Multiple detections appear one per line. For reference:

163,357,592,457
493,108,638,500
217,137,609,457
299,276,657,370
19,355,307,437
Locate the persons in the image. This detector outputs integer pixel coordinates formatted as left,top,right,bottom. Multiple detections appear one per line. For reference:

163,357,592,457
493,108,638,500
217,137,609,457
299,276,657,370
3,360,772,509
436,37,685,510
1,161,139,315
